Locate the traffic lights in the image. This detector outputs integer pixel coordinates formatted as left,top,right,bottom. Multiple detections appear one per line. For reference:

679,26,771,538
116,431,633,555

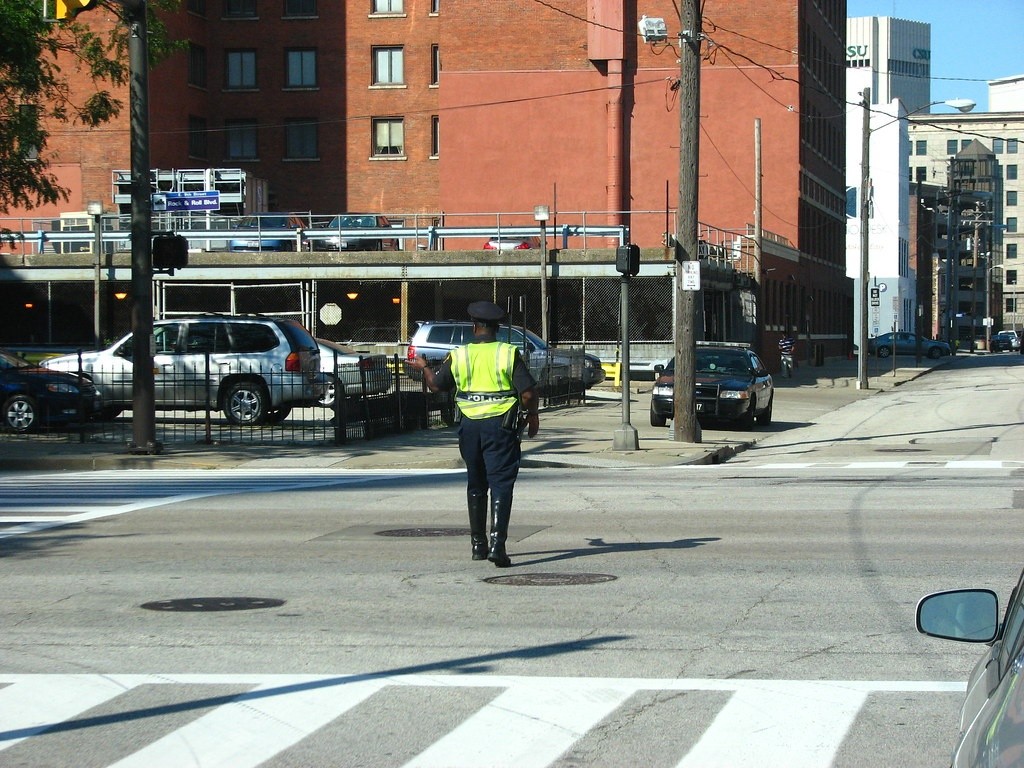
56,0,92,21
153,236,189,268
616,243,640,275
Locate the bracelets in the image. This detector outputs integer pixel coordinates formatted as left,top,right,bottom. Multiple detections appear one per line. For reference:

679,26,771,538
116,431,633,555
422,366,427,371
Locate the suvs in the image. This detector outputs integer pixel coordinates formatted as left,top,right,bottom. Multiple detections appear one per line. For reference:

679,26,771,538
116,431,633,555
408,321,607,404
37,311,323,425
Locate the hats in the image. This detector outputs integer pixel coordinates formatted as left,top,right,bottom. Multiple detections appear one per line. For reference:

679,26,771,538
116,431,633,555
468,300,505,327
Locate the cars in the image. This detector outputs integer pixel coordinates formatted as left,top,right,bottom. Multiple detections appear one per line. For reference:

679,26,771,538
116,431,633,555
483,236,542,250
869,331,952,359
970,330,1024,355
308,213,400,252
0,348,104,434
650,342,774,432
228,213,309,252
315,337,391,408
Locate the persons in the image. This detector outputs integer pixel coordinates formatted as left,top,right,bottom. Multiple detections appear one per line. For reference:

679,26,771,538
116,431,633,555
405,301,539,568
779,332,795,379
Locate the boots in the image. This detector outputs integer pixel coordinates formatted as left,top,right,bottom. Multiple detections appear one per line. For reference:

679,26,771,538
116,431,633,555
487,498,512,567
466,493,490,560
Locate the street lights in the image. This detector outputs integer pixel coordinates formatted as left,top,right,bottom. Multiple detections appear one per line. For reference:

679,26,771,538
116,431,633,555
946,225,1009,340
916,170,972,362
986,264,1004,351
1012,280,1016,331
855,96,976,390
